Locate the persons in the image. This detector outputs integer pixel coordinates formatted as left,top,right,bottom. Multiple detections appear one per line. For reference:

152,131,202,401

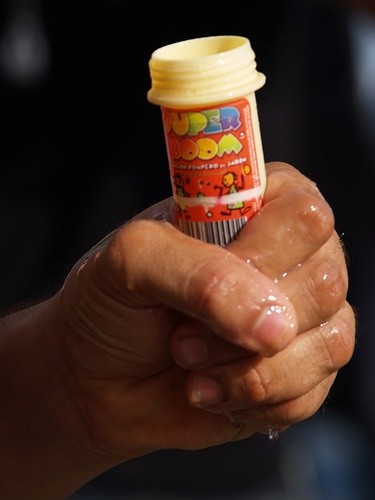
0,159,355,500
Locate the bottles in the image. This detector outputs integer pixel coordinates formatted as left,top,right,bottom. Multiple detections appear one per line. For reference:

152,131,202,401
146,35,267,246
